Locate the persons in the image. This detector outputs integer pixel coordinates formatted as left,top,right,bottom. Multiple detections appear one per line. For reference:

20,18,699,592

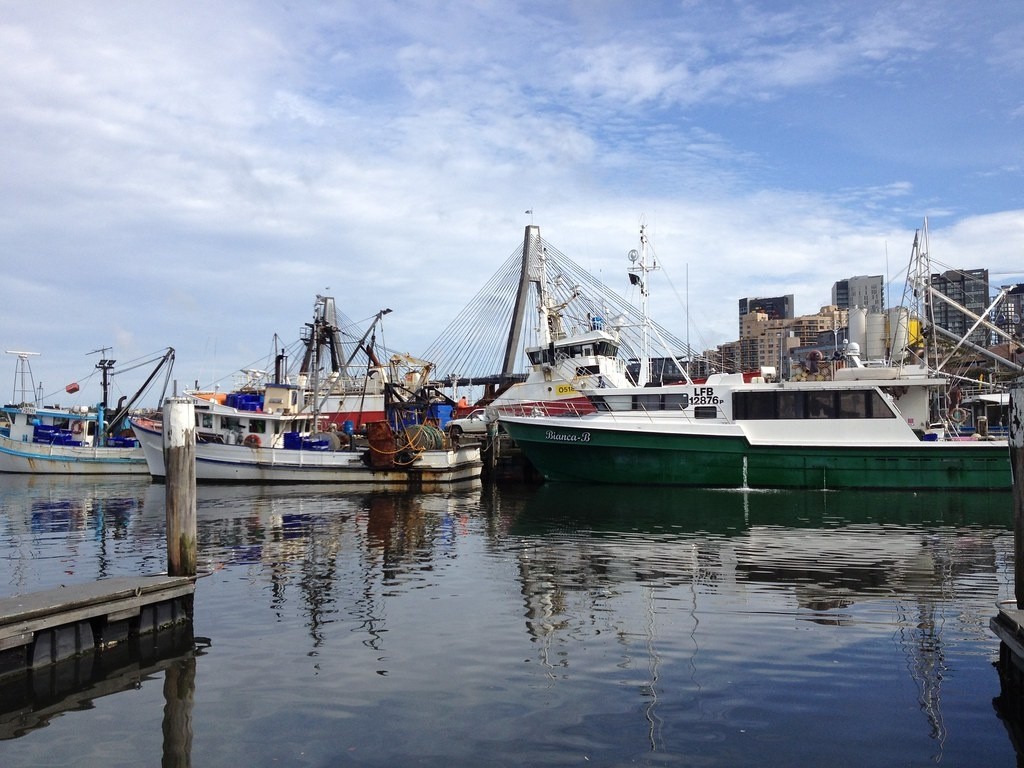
596,376,606,388
458,396,468,408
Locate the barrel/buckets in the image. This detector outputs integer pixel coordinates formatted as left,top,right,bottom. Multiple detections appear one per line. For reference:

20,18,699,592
107,437,140,447
343,419,353,435
284,432,329,451
921,434,937,441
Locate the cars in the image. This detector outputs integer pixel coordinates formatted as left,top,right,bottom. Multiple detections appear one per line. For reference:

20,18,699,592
444,407,507,435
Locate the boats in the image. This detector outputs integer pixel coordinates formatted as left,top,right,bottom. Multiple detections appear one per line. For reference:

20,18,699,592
490,213,1024,491
126,287,485,484
0,339,177,475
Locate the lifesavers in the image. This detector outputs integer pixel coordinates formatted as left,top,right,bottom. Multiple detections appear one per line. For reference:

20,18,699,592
995,315,1007,325
243,434,262,447
71,420,83,434
1012,314,1020,325
448,423,464,441
805,349,823,360
949,407,966,423
